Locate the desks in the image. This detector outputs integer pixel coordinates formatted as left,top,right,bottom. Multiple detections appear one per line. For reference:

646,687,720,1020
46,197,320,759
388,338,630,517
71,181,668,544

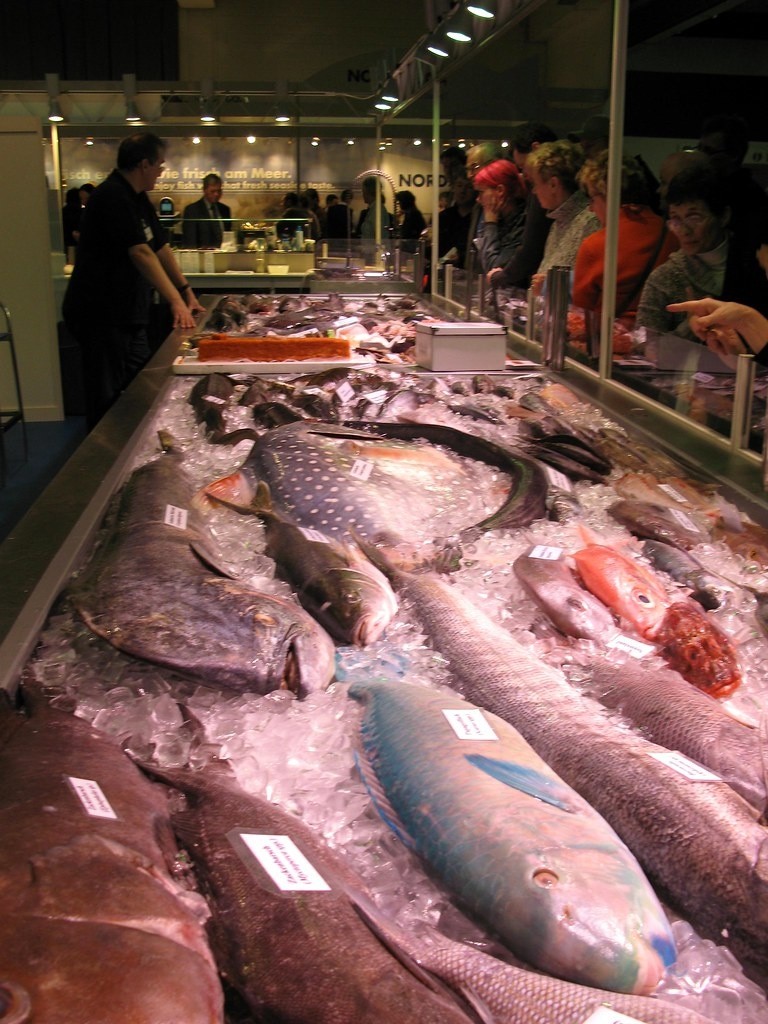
183,272,312,294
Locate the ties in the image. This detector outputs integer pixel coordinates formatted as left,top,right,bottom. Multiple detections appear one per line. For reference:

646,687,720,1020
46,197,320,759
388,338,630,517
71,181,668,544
210,204,221,230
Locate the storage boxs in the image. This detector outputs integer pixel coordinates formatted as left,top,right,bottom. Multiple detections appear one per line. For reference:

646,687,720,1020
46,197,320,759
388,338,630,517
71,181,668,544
416,321,508,372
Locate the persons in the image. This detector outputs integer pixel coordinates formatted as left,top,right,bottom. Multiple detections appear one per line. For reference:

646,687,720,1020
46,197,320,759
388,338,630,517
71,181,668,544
396,190,427,254
62,133,207,436
62,184,94,264
183,174,232,248
355,177,391,257
276,188,354,242
417,122,768,369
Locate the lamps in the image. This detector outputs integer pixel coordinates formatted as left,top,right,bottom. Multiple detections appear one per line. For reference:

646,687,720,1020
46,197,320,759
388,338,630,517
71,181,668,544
48,101,64,121
124,100,141,121
275,102,291,122
200,101,216,122
374,90,392,110
415,31,436,65
445,4,473,43
382,79,399,102
426,23,450,59
466,0,497,18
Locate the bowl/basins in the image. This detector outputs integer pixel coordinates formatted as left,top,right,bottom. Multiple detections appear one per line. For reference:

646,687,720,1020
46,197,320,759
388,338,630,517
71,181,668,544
266,265,289,274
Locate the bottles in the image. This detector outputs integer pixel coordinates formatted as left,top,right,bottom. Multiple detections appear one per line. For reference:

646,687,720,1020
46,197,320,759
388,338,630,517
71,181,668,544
296,226,303,247
281,228,289,250
256,238,265,273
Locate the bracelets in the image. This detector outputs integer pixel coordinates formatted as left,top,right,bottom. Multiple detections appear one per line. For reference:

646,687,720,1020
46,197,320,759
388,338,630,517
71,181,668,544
178,283,191,292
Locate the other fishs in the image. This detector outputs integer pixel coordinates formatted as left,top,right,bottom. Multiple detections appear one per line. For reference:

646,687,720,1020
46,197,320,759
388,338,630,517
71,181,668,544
194,362,642,551
119,724,490,1024
0,666,222,1024
572,662,768,809
218,416,480,569
244,484,394,644
358,534,768,969
66,430,334,696
579,474,768,696
208,290,436,368
388,922,717,1024
343,674,679,991
512,544,618,650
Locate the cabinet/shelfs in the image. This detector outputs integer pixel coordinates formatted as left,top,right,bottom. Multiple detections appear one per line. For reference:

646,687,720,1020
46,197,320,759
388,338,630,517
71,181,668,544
0,304,30,487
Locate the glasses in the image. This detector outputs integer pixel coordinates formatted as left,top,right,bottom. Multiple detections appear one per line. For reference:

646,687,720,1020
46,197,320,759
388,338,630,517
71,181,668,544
465,159,493,173
664,210,724,231
148,160,165,170
